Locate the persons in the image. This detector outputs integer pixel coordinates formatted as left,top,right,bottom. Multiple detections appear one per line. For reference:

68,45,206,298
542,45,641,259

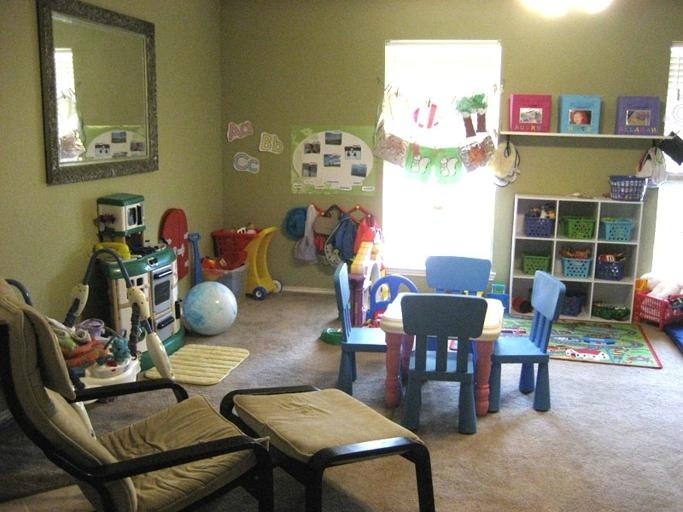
568,112,592,126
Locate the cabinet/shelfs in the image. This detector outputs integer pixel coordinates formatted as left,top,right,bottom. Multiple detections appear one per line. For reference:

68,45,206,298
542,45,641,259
509,194,644,324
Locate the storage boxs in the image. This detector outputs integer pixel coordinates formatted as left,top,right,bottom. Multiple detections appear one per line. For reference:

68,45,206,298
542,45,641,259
202,260,249,303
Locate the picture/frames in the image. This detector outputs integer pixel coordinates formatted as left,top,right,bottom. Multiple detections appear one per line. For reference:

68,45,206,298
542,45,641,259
613,95,660,136
558,95,601,134
509,94,552,132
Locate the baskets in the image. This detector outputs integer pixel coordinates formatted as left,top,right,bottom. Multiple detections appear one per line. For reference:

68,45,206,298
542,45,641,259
599,216,636,242
524,212,554,238
559,253,593,279
520,250,552,275
607,176,649,202
632,288,683,331
561,215,596,240
595,259,625,281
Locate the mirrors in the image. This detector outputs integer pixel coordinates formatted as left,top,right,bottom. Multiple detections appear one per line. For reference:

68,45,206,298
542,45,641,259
35,0,159,185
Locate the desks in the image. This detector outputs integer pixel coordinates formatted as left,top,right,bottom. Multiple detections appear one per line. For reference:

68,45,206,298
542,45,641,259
384,293,504,416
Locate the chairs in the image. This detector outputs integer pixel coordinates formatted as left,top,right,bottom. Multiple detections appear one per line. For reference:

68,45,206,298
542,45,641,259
489,271,567,413
400,295,487,434
426,257,493,297
0,275,276,512
335,262,387,397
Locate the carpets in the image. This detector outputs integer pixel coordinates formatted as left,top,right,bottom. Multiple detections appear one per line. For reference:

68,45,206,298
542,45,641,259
145,343,250,386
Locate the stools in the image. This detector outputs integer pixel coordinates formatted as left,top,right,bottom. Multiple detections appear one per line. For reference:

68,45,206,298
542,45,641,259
219,384,435,512
